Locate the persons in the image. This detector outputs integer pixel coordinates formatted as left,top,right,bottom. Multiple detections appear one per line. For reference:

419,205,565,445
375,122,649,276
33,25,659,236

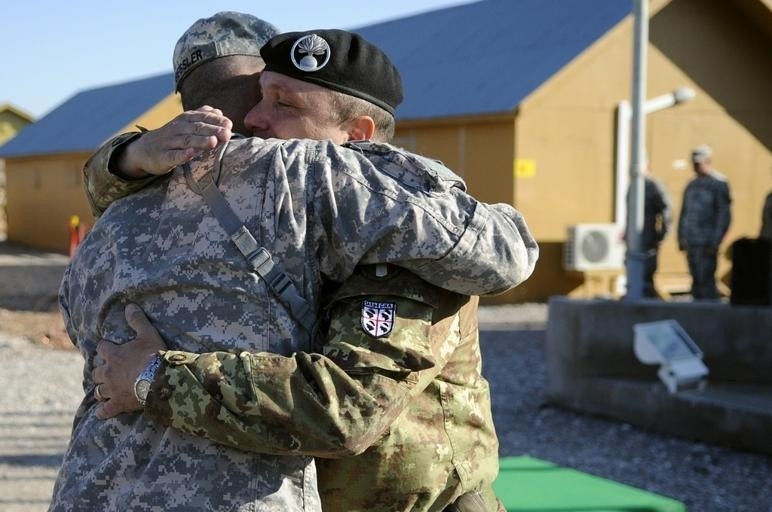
47,11,539,512
678,145,731,299
623,160,674,298
82,29,504,512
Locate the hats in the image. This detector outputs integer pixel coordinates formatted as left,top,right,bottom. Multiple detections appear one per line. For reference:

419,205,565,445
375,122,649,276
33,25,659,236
693,147,710,163
173,11,279,94
260,29,403,118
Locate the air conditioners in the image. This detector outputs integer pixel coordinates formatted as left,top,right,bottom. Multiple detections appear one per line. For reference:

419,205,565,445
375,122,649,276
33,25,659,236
565,221,624,274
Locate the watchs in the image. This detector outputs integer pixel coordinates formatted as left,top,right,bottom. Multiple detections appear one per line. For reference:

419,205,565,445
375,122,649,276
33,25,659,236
134,351,164,410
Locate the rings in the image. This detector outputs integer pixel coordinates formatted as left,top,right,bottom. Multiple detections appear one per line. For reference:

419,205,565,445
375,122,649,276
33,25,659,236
96,386,106,403
